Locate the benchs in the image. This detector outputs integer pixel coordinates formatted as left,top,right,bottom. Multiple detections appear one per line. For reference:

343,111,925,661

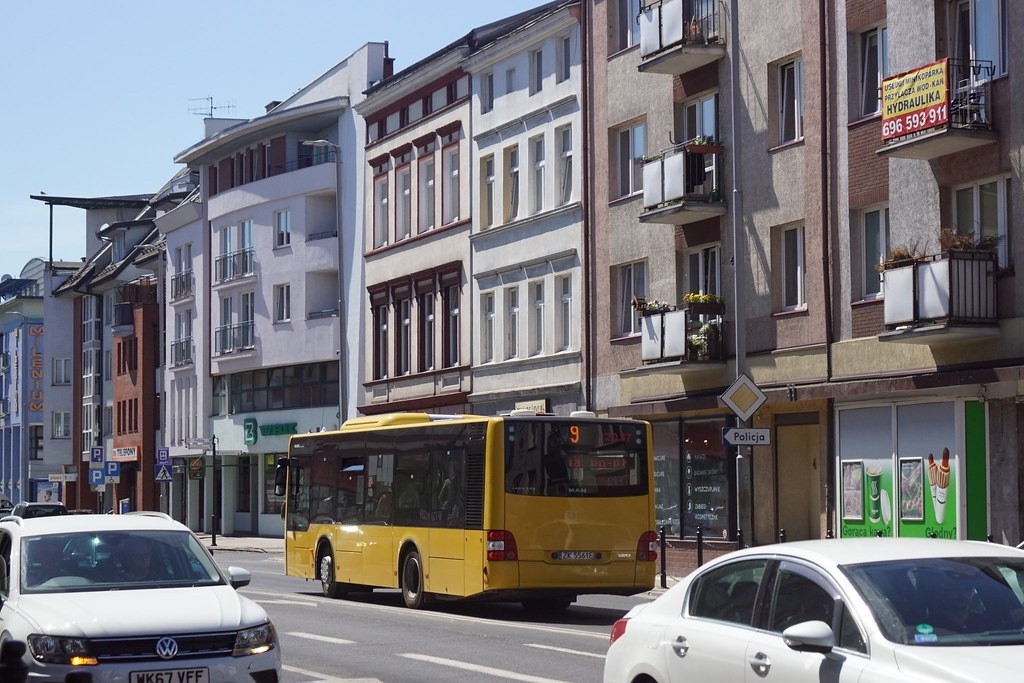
730,575,804,629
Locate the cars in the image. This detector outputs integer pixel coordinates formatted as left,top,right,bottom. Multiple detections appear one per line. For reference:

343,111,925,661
602,538,1024,683
275,412,657,610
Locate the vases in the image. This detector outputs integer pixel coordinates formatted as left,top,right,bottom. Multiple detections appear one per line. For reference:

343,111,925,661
688,350,699,361
688,302,723,315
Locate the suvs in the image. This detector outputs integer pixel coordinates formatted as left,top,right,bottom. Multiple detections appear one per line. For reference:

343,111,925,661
0,492,282,683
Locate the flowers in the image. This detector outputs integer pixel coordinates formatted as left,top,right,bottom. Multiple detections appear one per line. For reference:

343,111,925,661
687,334,708,352
682,291,728,304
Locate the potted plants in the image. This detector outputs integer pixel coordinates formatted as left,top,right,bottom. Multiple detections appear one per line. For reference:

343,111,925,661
685,135,724,155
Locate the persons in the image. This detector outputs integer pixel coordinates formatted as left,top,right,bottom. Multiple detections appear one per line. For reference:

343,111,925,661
112,542,163,581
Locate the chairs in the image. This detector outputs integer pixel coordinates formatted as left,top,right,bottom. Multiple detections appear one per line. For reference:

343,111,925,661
951,79,986,125
911,570,942,626
